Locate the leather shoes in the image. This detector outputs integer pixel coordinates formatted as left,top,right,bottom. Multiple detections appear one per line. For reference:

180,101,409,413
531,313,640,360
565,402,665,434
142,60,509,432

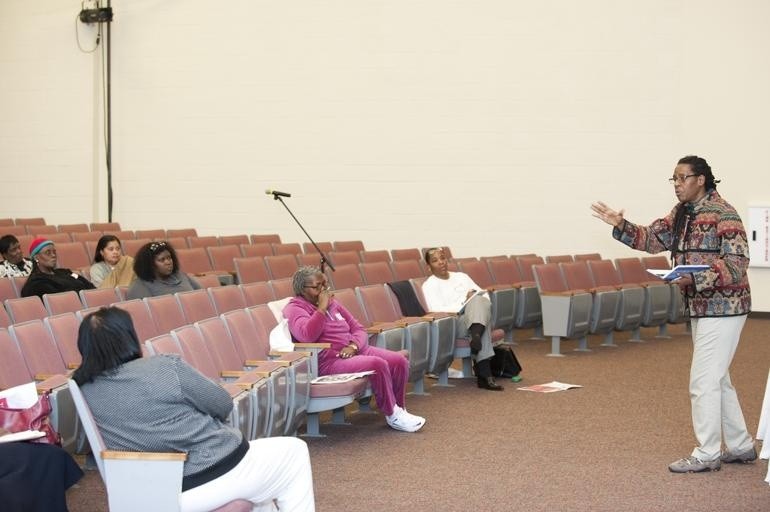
470,335,482,355
477,376,503,389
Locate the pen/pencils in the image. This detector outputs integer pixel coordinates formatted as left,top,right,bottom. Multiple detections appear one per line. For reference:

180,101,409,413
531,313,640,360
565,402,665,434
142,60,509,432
315,286,331,305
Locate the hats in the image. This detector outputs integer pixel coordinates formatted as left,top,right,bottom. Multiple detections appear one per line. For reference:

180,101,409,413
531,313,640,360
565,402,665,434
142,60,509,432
29,239,53,259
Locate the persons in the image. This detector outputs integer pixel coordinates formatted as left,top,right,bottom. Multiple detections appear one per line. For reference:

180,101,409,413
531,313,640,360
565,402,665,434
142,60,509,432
91,236,138,289
281,264,427,432
22,238,96,296
590,156,758,474
422,247,506,390
124,241,202,299
70,306,315,512
1,234,34,277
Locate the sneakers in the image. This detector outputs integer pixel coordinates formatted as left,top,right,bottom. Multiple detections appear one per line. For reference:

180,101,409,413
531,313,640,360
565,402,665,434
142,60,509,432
721,448,756,463
668,456,722,473
386,403,426,432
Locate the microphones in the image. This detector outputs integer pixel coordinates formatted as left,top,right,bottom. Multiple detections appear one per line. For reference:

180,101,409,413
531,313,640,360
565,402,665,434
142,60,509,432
266,188,291,197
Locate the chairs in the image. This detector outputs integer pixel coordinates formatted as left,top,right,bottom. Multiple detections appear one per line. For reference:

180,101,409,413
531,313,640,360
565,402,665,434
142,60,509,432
1,217,690,512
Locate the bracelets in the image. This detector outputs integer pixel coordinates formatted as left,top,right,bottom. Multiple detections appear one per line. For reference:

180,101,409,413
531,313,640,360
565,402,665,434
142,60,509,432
348,343,359,355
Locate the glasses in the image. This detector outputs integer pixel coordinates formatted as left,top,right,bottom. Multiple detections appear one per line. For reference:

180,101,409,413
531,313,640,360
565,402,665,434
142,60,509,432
151,242,166,251
670,174,700,184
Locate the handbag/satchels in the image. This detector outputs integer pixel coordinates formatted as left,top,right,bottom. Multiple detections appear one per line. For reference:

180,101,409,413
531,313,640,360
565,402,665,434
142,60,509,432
0,391,59,444
490,347,522,378
